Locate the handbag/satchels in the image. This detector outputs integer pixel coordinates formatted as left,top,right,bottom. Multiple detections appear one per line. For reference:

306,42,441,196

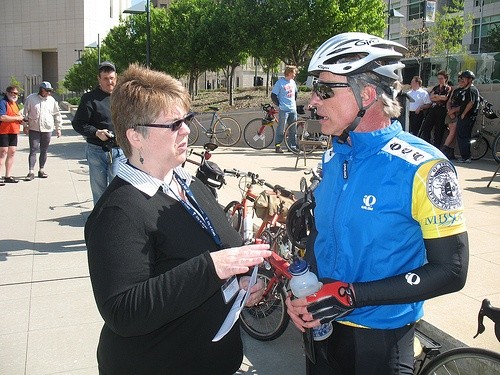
253,190,279,221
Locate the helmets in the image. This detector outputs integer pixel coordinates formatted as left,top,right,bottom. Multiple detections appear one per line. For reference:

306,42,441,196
461,70,475,80
485,109,498,119
307,32,411,83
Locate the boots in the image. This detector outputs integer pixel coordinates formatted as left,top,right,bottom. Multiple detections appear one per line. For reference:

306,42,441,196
441,144,458,160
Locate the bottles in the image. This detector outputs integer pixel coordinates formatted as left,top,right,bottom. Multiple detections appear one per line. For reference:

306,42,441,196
289,255,333,341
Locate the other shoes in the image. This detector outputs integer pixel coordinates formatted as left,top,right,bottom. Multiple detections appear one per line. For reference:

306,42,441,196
4,176,18,183
456,157,472,163
0,176,6,186
288,147,299,152
276,146,284,153
38,171,48,177
25,173,34,181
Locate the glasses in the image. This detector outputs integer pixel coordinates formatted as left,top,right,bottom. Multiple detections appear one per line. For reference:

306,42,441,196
11,92,18,95
312,81,351,101
131,112,197,132
44,88,51,92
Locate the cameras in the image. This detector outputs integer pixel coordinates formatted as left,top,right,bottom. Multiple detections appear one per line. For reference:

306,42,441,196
102,131,117,152
19,115,30,122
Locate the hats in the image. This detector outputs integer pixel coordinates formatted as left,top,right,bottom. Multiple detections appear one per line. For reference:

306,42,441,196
40,81,54,90
98,61,116,72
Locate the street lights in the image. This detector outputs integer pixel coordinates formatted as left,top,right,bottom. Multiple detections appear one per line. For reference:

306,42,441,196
122,0,150,70
84,33,100,65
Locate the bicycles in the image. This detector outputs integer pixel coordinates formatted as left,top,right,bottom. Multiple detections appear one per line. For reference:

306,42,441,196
469,95,500,164
181,141,324,341
186,106,242,147
243,103,334,156
413,298,500,375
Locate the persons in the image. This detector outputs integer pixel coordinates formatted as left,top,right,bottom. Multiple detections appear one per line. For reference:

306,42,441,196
22,81,63,181
85,62,272,375
72,60,126,208
405,75,432,137
392,81,405,131
443,72,467,160
0,85,25,186
271,65,301,153
456,71,479,163
279,32,469,375
423,69,452,149
